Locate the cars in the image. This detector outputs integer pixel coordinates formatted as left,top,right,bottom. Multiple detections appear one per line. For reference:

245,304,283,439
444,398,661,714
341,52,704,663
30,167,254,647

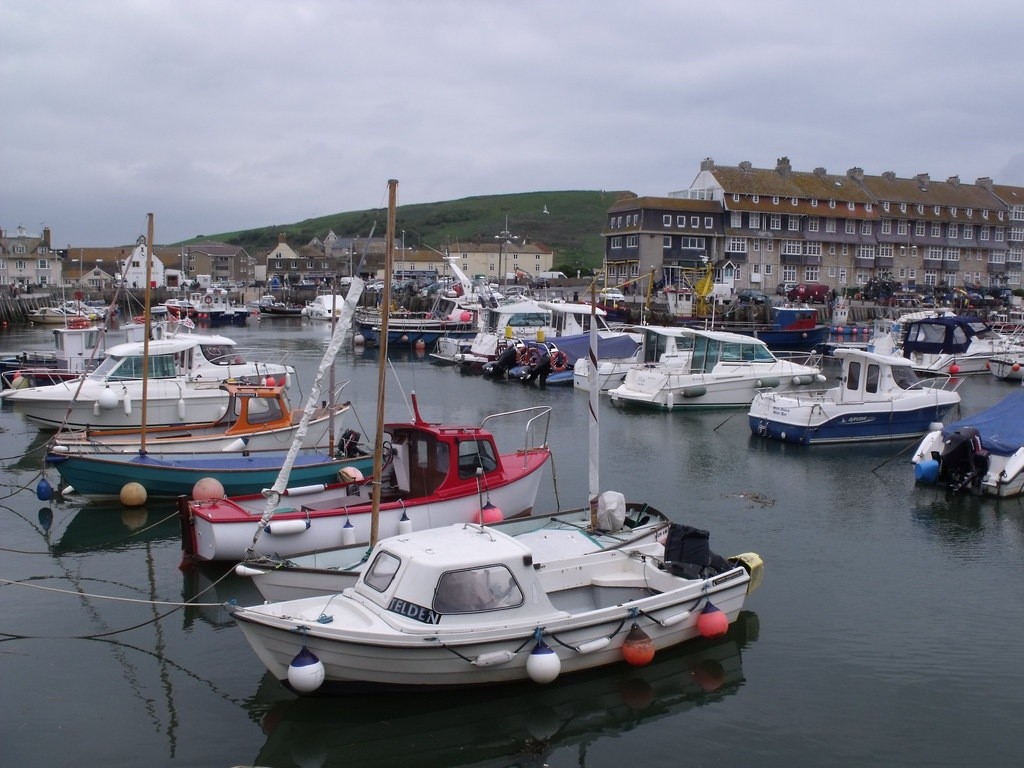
364,278,529,297
599,287,625,303
527,277,550,289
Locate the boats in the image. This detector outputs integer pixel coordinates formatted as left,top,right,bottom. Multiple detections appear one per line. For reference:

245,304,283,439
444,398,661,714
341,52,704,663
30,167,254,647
176,388,552,561
357,208,824,410
25,296,120,323
223,179,762,693
817,308,1024,382
911,388,1024,497
0,307,388,506
748,348,967,447
157,285,351,325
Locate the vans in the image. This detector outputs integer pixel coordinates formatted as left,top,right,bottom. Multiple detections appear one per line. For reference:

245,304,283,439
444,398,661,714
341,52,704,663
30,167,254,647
539,272,567,278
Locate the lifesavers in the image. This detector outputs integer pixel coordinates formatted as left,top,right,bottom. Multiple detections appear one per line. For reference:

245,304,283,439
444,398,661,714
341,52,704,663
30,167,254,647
550,351,568,371
66,325,90,328
66,316,88,321
204,295,212,304
516,347,527,365
65,321,92,326
527,348,542,365
